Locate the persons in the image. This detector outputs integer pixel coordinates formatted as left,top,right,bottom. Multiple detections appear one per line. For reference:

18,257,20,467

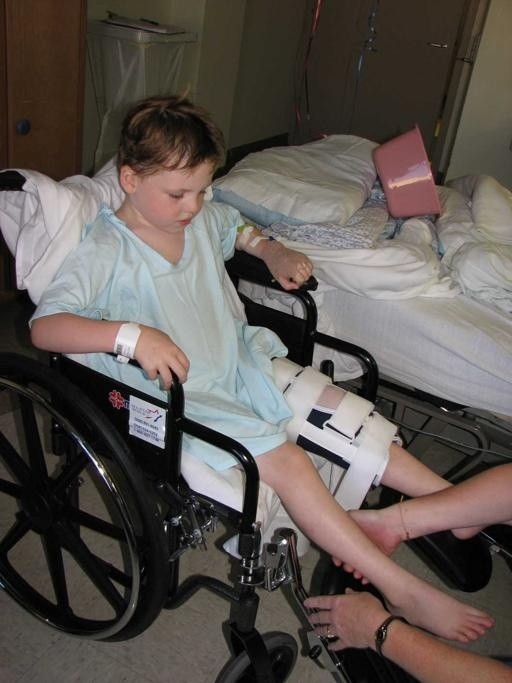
29,95,497,644
302,463,511,681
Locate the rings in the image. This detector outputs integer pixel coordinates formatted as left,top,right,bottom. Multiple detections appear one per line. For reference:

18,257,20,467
326,625,335,639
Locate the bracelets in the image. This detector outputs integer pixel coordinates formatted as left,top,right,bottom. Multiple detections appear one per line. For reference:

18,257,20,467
400,496,412,543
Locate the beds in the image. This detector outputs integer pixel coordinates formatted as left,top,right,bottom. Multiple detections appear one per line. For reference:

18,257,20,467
209,133,512,484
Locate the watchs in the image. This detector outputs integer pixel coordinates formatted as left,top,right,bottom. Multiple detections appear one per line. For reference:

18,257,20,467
374,613,406,658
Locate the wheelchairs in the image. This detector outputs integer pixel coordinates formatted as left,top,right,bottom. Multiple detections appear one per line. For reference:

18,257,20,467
0,168,496,680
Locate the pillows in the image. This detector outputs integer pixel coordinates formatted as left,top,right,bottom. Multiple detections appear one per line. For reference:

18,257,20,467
212,126,378,230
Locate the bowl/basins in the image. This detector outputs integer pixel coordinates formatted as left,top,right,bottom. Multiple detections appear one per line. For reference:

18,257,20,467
370,124,442,218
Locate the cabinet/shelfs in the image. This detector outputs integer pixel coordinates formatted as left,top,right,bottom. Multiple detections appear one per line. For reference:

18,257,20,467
0,0,89,301
